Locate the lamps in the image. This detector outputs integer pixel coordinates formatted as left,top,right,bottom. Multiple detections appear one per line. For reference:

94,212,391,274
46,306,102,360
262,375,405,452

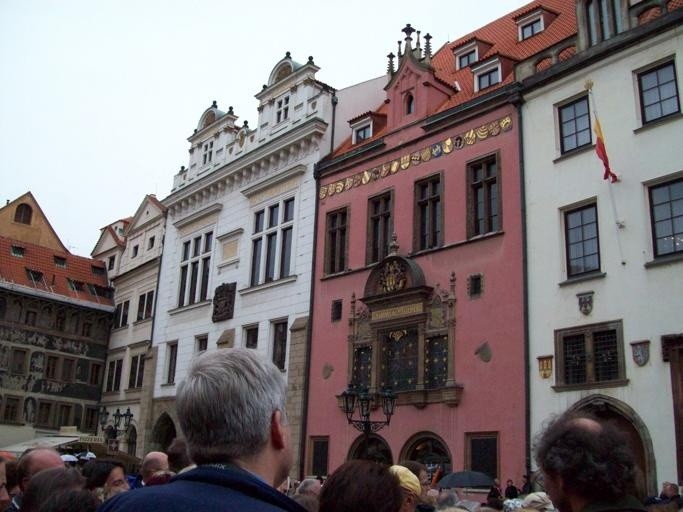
98,406,133,433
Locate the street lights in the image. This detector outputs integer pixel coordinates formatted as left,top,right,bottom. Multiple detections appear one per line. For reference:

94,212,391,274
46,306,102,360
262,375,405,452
339,384,399,458
97,405,134,440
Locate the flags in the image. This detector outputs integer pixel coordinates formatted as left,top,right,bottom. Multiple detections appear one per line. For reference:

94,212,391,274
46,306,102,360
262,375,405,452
594,113,616,183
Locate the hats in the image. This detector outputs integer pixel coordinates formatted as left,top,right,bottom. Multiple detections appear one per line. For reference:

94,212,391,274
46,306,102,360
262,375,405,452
386,463,430,506
520,490,552,509
